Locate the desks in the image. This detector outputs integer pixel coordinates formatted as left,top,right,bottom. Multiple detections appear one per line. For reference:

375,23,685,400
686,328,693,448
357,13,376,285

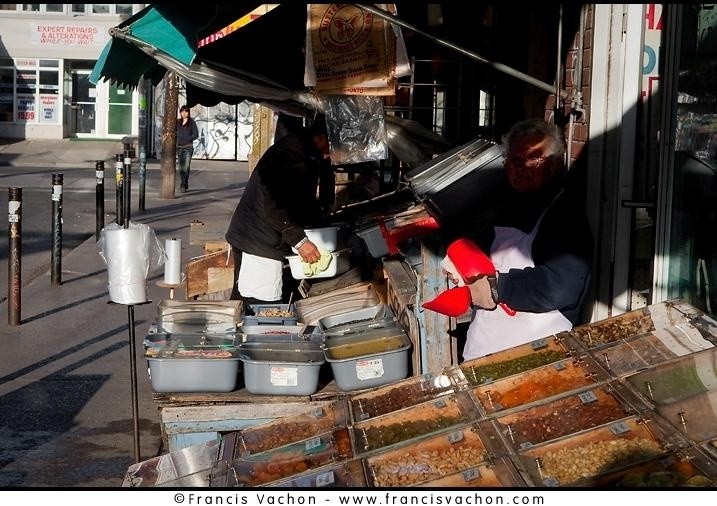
156,391,330,454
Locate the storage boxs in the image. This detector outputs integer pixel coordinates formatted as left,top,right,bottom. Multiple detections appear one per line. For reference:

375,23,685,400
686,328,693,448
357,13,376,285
290,223,346,253
283,247,352,282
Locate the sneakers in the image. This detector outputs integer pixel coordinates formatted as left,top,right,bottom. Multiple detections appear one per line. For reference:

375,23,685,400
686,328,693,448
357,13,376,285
180,184,189,193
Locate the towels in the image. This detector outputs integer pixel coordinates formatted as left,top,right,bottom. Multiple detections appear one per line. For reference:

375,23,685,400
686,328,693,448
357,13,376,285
299,246,333,278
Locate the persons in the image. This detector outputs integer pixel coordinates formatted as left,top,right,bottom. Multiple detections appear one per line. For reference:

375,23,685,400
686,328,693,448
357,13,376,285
425,119,597,361
223,119,336,308
174,105,198,192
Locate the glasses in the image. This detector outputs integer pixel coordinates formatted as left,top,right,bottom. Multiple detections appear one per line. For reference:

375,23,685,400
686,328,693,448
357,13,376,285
501,154,549,170
